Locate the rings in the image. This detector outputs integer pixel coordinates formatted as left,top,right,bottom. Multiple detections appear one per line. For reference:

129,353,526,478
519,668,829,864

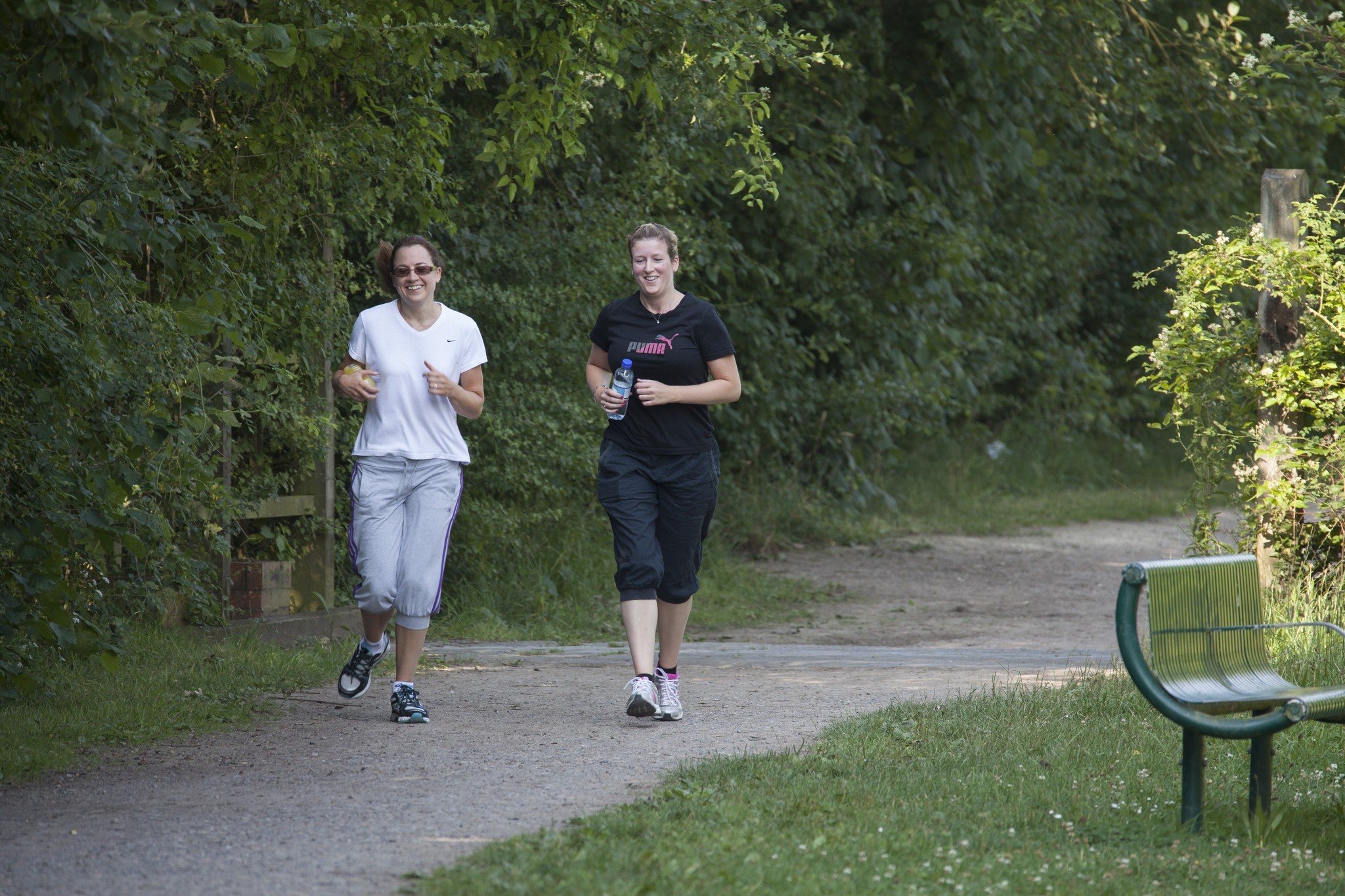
435,383,438,389
603,404,605,407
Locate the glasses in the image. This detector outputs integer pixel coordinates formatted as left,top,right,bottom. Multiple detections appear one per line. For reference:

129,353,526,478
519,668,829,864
395,265,438,277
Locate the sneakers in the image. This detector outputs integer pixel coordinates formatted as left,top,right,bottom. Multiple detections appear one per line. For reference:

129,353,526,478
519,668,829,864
622,676,662,717
390,684,429,724
337,631,390,698
653,659,683,721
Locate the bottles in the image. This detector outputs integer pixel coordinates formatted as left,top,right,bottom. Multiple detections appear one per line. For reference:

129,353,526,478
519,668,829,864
605,359,634,420
344,364,375,389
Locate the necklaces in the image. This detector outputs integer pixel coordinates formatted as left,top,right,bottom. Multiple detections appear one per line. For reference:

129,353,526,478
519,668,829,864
641,296,673,324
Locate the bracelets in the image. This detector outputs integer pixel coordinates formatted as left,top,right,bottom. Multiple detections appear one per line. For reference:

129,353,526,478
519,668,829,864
593,385,609,404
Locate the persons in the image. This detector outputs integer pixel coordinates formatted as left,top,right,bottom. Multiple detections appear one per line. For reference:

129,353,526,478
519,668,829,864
586,222,741,723
338,234,489,723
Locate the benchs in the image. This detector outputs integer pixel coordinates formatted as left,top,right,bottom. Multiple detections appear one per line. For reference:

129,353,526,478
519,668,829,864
1115,554,1345,834
